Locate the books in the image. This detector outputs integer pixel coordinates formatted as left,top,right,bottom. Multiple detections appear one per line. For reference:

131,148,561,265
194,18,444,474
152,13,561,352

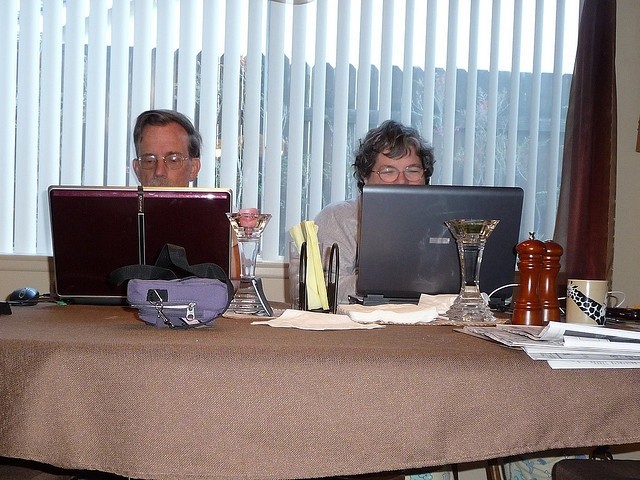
467,320,640,369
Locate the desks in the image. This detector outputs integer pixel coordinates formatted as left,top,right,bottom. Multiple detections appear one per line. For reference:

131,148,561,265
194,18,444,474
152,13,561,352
1,299,640,479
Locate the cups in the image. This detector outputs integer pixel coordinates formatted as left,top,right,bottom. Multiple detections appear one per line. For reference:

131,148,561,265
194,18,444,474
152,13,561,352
566,278,627,326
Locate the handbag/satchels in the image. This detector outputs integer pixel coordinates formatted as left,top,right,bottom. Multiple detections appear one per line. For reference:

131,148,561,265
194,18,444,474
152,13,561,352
110,243,234,328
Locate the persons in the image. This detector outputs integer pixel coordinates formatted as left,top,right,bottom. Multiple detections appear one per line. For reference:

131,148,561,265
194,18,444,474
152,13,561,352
315,120,436,304
132,109,201,188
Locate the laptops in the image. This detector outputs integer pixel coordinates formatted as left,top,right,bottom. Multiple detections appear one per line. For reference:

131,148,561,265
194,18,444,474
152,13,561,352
347,185,524,312
48,186,232,306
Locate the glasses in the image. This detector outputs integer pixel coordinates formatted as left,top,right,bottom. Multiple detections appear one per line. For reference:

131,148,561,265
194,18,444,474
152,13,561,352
367,163,426,182
136,153,194,170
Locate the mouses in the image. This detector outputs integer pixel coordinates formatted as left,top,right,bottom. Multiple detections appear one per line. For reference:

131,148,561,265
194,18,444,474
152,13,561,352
11,287,39,305
